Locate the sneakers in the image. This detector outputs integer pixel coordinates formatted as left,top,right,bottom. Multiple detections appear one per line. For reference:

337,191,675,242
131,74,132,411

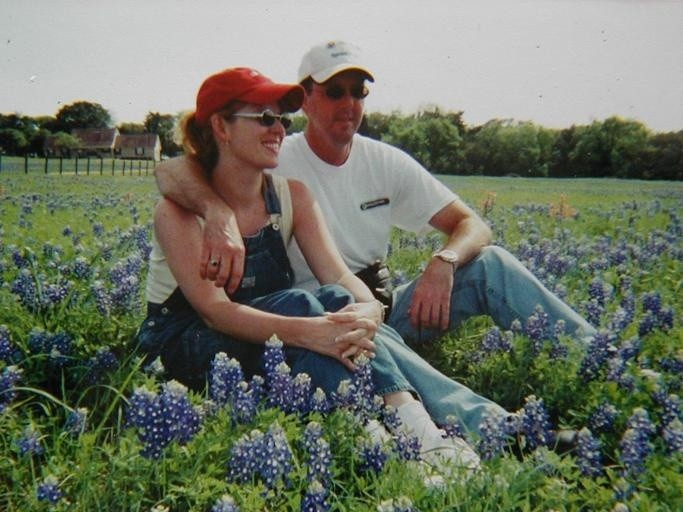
414,461,446,491
547,429,578,450
434,436,480,481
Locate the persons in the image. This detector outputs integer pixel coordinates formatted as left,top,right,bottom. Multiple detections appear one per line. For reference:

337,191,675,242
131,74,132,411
152,39,663,469
134,68,482,493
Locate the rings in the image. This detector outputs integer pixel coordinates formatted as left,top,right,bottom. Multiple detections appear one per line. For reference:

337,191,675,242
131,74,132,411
210,260,219,266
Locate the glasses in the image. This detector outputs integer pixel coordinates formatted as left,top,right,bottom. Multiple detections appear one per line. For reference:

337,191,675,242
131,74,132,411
313,85,368,98
232,112,292,129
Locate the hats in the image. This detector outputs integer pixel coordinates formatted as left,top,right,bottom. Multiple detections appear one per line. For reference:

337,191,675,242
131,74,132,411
299,40,374,83
195,68,304,124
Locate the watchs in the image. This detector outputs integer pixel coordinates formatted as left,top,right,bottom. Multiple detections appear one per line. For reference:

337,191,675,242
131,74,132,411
433,249,458,274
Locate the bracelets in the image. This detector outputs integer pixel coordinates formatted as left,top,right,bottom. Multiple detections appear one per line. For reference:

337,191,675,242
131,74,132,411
373,300,385,323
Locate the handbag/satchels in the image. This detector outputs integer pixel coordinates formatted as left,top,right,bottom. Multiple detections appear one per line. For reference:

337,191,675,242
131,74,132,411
354,260,393,321
